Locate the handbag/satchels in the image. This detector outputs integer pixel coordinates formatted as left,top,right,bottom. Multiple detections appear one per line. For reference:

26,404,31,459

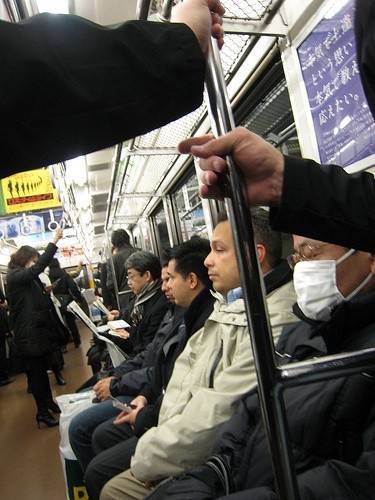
55,295,74,309
54,391,102,500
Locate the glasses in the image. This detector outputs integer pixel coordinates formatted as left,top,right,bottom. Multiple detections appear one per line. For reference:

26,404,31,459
126,272,140,279
286,243,330,270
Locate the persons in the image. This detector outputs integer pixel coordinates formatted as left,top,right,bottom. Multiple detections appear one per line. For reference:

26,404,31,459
89,284,104,343
0,283,16,387
143,234,375,500
100,245,120,311
6,227,73,429
68,246,186,500
38,272,65,373
0,0,226,180
48,257,83,353
23,300,66,394
106,228,142,311
87,293,137,377
178,0,375,255
74,249,168,393
85,238,216,500
67,258,83,278
99,203,300,500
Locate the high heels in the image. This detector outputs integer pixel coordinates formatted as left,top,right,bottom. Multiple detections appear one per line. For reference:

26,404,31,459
54,372,66,385
48,401,62,413
35,411,59,429
27,383,33,393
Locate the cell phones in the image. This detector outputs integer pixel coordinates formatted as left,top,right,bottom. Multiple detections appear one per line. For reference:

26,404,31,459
51,279,61,286
108,394,137,410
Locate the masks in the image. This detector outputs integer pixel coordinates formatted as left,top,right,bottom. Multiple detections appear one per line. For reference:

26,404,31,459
293,249,373,322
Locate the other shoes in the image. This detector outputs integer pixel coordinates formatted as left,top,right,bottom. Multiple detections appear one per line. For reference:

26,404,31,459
0,376,16,386
